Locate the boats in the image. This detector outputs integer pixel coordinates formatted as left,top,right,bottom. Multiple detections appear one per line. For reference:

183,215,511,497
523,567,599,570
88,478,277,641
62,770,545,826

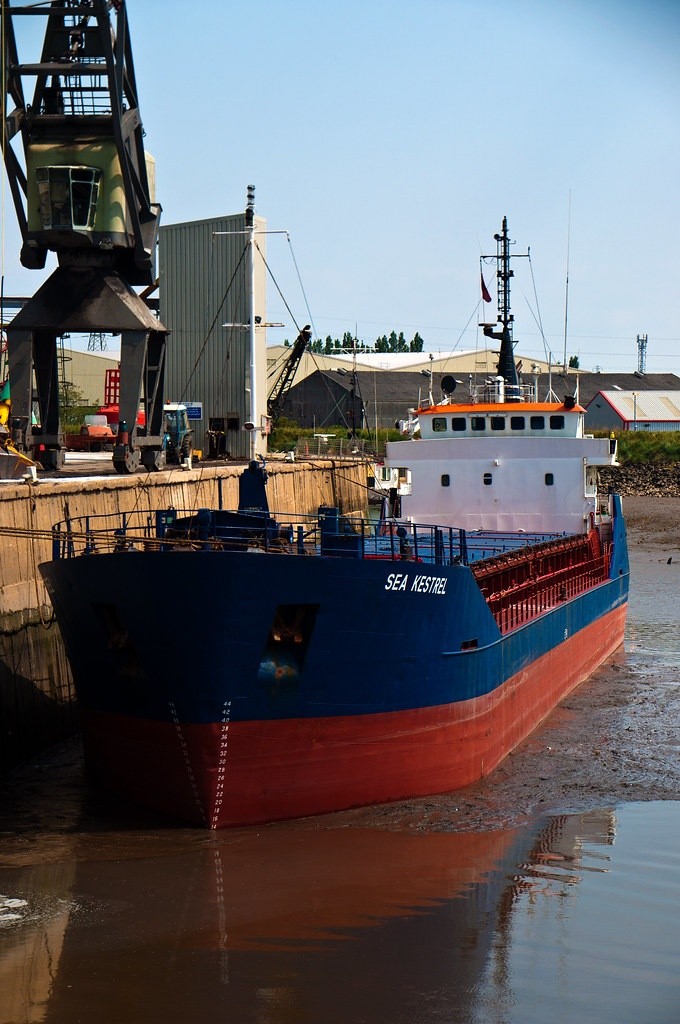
32,213,634,834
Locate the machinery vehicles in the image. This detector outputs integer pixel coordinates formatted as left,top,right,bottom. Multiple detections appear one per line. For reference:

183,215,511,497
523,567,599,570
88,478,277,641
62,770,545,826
162,399,204,465
